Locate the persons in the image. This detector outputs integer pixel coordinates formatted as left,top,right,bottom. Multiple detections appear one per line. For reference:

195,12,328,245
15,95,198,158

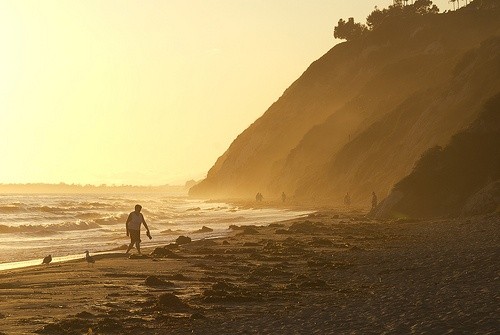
256,193,264,202
126,205,150,254
372,192,377,208
282,192,286,201
344,192,350,207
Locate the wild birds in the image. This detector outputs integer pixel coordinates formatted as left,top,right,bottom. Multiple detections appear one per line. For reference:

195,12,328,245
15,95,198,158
42,255,52,265
84,250,95,268
